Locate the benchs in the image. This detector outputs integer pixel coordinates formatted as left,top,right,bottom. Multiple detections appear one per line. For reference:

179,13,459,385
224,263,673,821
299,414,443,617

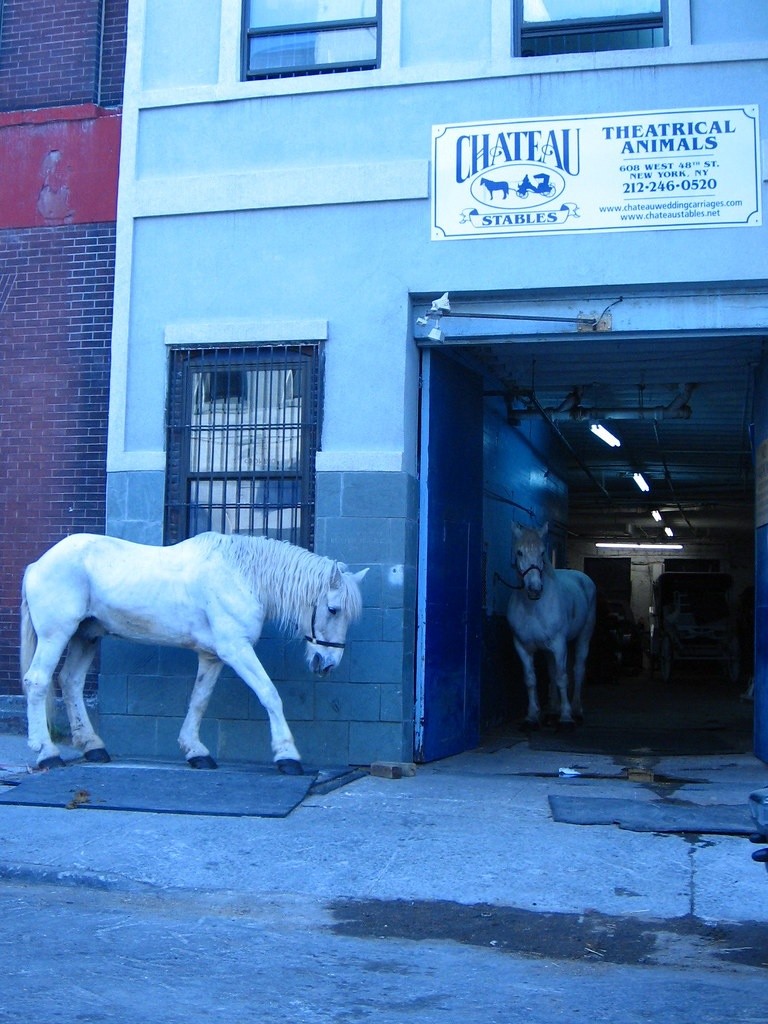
668,620,729,637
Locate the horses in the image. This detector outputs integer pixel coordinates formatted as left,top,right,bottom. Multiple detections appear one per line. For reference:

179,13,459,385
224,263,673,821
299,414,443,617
20,531,371,776
506,519,597,727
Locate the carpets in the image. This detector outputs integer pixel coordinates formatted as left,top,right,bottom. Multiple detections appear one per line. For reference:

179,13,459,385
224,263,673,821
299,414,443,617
547,792,756,838
0,766,318,820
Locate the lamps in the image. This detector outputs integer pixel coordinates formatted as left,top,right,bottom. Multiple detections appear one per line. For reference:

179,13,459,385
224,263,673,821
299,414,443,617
414,293,601,347
589,420,622,449
